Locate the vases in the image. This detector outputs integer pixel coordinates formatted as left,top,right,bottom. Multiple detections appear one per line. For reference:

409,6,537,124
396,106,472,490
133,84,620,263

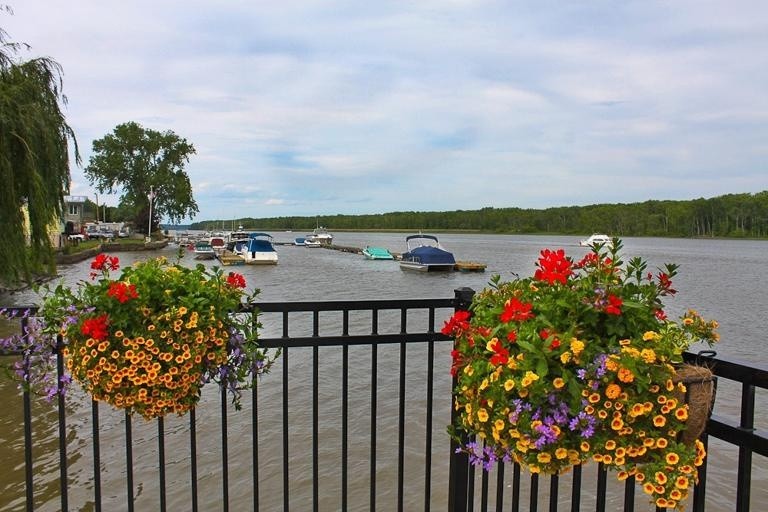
668,350,717,446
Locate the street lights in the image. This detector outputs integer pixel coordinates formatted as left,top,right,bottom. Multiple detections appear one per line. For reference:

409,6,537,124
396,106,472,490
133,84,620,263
148,182,156,242
94,193,99,222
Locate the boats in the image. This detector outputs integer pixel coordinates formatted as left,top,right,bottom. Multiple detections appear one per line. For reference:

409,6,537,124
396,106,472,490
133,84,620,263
174,213,278,267
361,246,394,262
295,218,333,248
579,234,616,247
399,233,457,274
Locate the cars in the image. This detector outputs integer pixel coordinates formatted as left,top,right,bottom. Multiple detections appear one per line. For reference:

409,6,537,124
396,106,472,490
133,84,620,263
66,223,131,243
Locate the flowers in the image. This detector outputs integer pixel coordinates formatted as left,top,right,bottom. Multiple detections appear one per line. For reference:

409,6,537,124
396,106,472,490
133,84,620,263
442,238,719,512
